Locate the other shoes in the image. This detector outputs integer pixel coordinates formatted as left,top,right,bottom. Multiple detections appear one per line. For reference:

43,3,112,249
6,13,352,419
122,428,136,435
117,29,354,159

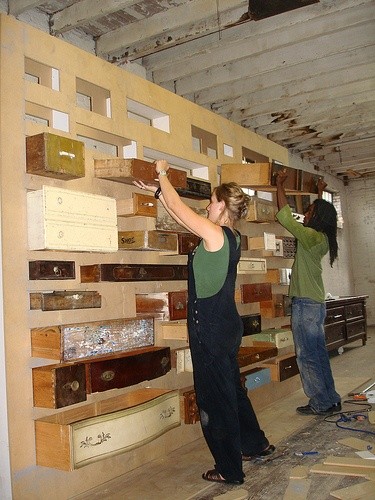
332,402,341,412
297,404,333,416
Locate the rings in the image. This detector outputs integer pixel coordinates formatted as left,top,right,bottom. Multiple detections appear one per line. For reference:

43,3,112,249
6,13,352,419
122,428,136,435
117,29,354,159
141,186,143,189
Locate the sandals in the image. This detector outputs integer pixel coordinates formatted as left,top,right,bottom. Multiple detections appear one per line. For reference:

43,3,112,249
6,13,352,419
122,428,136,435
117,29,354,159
202,470,244,484
241,445,275,461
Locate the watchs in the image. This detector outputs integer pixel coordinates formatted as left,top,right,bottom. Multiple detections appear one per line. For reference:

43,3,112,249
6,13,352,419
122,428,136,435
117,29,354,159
157,170,167,176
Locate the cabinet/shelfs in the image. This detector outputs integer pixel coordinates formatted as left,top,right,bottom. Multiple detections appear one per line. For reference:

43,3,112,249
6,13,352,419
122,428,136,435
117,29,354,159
325,294,369,349
0,12,325,500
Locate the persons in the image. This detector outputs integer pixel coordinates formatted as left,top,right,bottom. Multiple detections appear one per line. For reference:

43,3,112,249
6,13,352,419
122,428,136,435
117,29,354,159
132,159,277,485
276,172,343,417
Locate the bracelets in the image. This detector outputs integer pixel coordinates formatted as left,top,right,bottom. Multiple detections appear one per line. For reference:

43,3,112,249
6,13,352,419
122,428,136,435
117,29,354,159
155,187,161,199
